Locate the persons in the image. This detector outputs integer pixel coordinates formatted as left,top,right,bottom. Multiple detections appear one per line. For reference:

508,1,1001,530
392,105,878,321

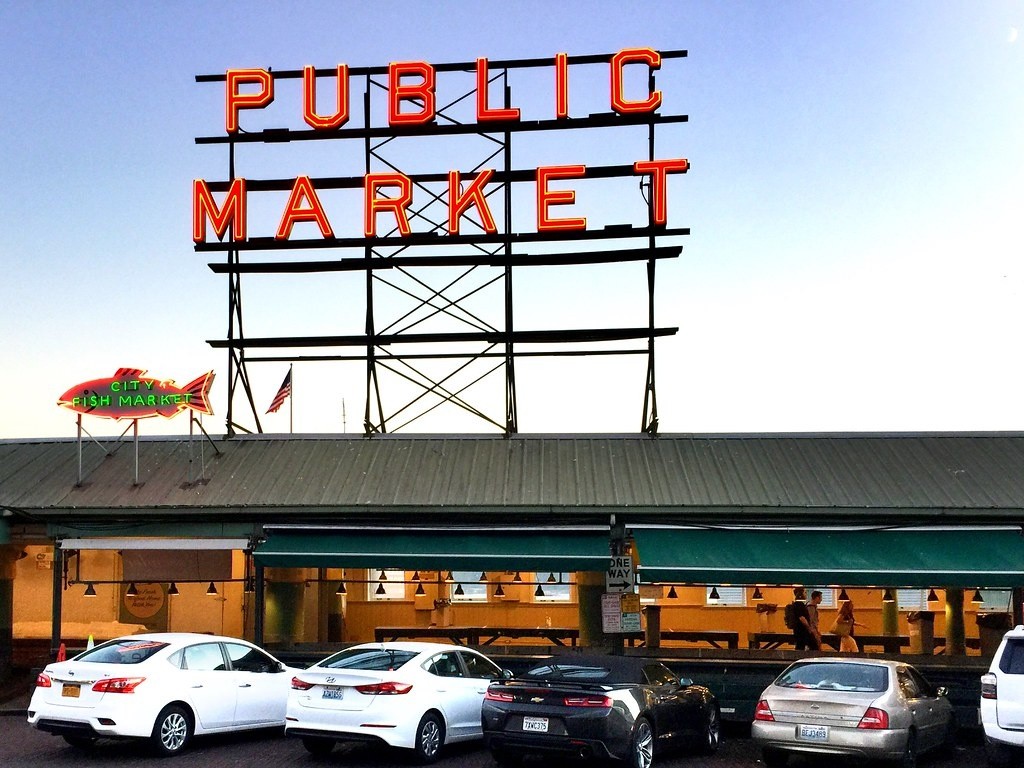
788,588,820,651
803,591,824,651
835,599,870,653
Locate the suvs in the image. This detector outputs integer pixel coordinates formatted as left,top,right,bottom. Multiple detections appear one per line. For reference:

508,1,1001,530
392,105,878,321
976,626,1023,759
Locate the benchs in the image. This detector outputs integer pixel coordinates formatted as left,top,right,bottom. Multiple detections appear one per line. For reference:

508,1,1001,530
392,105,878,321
747,632,980,657
374,626,739,651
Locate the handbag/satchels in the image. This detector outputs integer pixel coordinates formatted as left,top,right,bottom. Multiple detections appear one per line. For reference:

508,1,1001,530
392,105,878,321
829,612,854,638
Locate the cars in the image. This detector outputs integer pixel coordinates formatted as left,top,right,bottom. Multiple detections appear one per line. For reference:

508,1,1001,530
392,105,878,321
284,640,517,758
26,633,306,753
749,657,957,767
479,652,720,768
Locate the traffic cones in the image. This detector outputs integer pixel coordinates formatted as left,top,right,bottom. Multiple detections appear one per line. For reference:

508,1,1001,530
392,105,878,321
56,643,66,663
86,635,94,652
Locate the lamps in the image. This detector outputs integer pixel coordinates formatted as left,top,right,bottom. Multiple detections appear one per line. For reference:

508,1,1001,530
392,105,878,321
206,581,218,595
837,588,851,602
751,587,765,601
83,582,98,598
708,586,721,600
926,588,939,603
333,567,560,599
242,581,256,594
971,589,985,605
794,588,807,602
881,588,895,603
126,582,139,597
167,582,180,596
666,586,679,599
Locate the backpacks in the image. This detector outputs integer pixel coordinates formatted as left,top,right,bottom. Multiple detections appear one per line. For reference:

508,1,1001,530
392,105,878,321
784,601,797,629
